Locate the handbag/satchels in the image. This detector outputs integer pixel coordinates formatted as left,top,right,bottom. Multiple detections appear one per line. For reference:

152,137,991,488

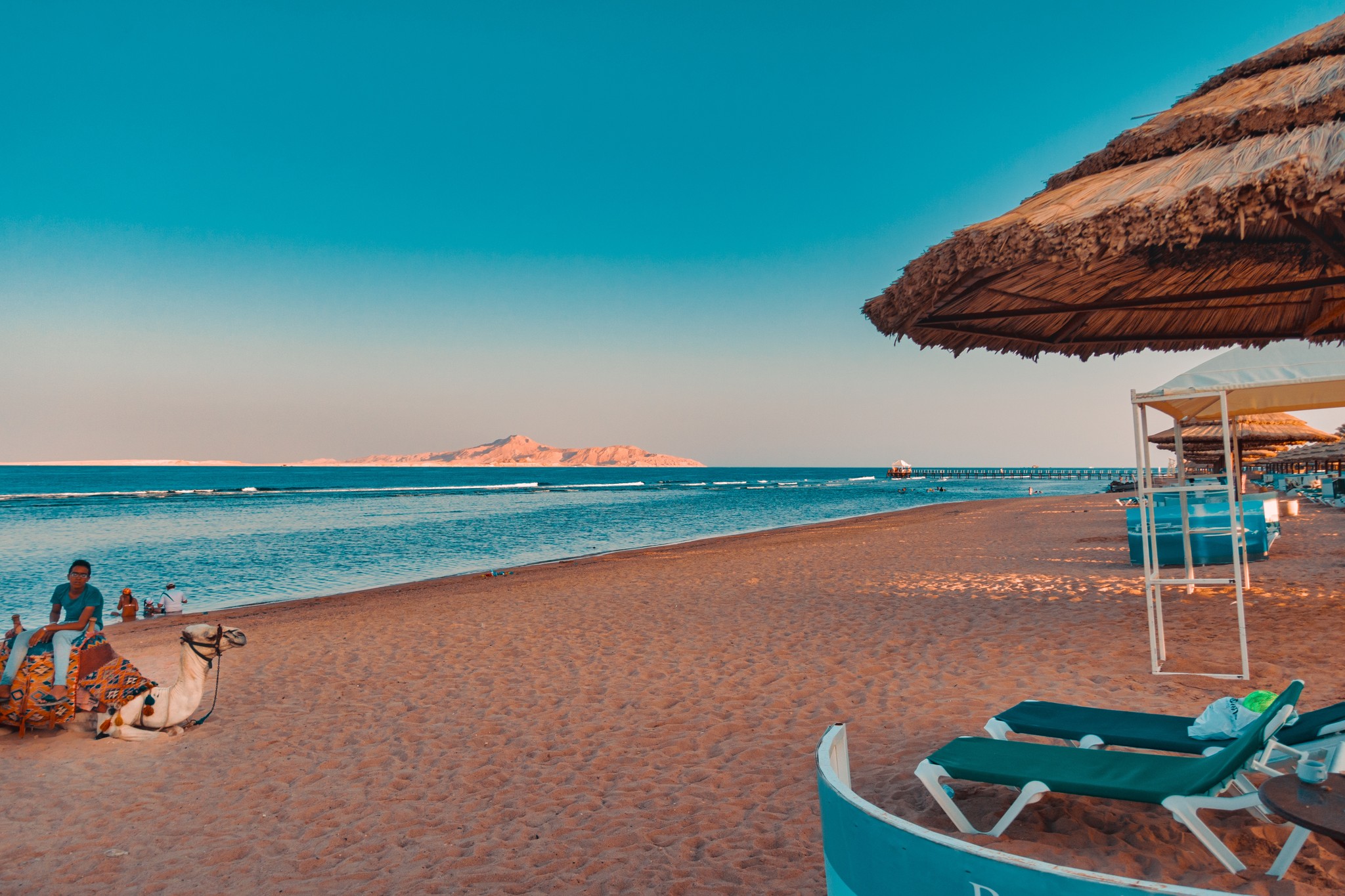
1188,696,1300,741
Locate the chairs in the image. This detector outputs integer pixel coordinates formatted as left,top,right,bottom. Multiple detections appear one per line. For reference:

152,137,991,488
914,679,1310,875
1115,495,1148,507
985,698,1345,826
1250,480,1345,508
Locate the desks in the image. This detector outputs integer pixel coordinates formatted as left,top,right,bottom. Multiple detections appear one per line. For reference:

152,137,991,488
1257,773,1345,851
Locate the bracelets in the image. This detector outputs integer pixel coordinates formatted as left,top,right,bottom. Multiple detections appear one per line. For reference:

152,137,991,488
41,626,48,633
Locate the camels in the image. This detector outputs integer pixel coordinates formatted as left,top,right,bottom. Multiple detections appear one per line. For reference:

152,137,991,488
0,623,249,746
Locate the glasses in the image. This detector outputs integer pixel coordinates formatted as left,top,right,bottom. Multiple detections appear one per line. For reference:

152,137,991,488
68,572,90,578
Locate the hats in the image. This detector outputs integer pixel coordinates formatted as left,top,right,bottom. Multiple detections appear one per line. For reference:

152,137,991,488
122,588,131,594
146,597,154,604
167,581,175,586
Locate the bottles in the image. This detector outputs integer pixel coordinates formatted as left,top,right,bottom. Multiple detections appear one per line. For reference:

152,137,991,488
941,785,954,799
203,612,208,614
508,572,514,574
500,571,505,575
491,568,494,576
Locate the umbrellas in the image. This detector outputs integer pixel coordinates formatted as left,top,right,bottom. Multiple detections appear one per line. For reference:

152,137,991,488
1241,439,1345,477
1145,411,1340,482
861,13,1344,364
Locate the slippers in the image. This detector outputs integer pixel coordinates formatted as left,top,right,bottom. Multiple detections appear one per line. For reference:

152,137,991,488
34,694,68,706
0,697,10,702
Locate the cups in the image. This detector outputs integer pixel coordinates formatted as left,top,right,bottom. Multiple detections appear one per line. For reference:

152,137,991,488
1297,760,1327,783
575,563,577,564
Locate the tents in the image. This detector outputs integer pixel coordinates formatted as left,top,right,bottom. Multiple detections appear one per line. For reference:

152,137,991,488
1130,337,1345,681
892,460,911,473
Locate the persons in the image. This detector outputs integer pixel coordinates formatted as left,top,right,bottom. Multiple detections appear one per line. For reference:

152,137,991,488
926,486,946,492
1034,491,1038,493
898,487,913,491
1120,477,1123,482
143,597,155,617
890,467,911,473
0,559,104,708
117,588,139,617
1125,476,1128,482
1039,490,1042,493
156,581,188,616
